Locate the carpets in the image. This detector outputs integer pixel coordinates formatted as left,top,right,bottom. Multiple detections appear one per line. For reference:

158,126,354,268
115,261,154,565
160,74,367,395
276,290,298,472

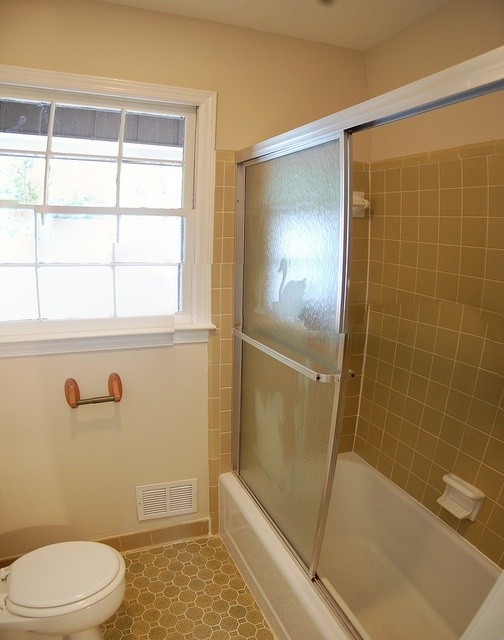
97,535,276,640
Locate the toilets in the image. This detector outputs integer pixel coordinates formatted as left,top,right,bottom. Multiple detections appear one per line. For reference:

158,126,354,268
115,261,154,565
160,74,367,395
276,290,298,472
0,540,127,640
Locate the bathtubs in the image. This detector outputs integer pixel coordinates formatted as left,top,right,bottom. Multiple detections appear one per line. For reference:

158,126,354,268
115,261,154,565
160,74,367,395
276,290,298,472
219,451,504,640
